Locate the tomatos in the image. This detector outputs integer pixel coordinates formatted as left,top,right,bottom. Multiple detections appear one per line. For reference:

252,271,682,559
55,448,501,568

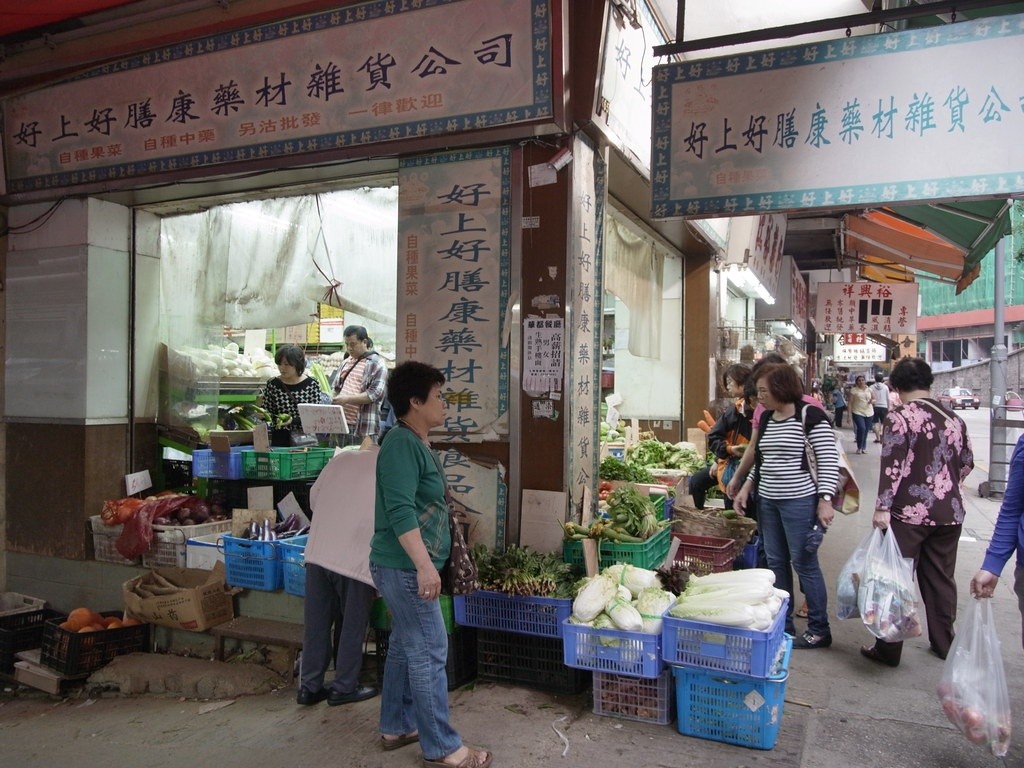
598,481,612,500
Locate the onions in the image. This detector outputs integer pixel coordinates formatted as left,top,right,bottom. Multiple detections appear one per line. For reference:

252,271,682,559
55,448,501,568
101,490,231,525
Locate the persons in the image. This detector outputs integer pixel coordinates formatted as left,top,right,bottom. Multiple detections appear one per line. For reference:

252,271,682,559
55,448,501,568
830,373,904,454
296,427,392,705
689,344,849,649
368,362,493,768
860,356,975,667
329,325,397,449
260,344,321,447
970,433,1024,648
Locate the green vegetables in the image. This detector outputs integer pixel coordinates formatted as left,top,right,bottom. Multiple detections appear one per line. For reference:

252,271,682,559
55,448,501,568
628,439,715,471
600,455,657,484
470,542,574,596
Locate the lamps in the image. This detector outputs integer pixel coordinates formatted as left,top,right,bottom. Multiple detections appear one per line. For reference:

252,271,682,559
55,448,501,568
722,248,751,270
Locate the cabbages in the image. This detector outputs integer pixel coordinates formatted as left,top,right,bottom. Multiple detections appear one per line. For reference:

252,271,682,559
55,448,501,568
569,561,677,669
669,568,790,660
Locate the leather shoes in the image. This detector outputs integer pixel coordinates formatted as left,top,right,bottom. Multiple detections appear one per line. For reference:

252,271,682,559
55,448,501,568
296,687,327,705
327,685,378,706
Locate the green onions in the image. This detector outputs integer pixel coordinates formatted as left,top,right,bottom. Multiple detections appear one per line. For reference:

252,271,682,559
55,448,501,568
606,485,657,537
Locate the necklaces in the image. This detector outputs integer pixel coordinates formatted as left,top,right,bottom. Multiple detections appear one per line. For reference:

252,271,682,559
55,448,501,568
401,418,431,447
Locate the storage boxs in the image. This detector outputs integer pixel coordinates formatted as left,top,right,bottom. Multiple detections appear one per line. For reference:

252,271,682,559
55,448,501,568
87,441,795,750
266,302,344,344
40,610,150,680
0,591,67,670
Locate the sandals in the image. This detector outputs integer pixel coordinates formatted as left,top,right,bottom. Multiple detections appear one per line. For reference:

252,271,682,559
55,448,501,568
381,734,419,749
423,743,493,768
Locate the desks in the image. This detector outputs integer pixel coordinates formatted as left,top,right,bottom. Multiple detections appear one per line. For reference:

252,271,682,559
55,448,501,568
150,618,303,684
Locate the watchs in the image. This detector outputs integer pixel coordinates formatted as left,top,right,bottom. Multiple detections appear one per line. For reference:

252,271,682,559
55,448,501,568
818,494,832,502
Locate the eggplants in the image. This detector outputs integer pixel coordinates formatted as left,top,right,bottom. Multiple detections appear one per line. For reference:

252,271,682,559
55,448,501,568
240,513,310,547
224,415,239,431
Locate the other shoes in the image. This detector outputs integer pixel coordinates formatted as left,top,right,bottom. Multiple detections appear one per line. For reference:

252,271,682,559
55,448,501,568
792,630,832,649
721,459,754,502
861,637,903,667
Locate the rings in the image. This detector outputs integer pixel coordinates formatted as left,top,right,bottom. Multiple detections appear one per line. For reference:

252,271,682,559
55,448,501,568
425,592,429,594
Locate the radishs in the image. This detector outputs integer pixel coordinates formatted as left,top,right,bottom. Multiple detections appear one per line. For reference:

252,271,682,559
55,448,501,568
181,341,259,377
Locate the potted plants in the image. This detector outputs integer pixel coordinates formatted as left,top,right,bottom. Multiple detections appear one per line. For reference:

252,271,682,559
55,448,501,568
602,334,615,356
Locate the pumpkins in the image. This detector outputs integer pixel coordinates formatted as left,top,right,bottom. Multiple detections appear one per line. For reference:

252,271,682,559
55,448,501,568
55,607,142,667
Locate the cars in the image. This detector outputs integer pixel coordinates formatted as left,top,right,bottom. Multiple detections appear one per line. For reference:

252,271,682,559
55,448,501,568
936,388,980,410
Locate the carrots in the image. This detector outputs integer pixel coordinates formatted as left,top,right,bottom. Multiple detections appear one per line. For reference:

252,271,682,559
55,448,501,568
697,410,716,433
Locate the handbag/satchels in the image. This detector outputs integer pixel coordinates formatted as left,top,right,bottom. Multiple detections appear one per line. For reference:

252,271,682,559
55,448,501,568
438,512,479,595
835,524,922,642
936,592,1011,757
802,404,849,496
834,432,860,514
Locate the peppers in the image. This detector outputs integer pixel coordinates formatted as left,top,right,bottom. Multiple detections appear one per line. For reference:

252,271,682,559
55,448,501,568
232,404,292,431
